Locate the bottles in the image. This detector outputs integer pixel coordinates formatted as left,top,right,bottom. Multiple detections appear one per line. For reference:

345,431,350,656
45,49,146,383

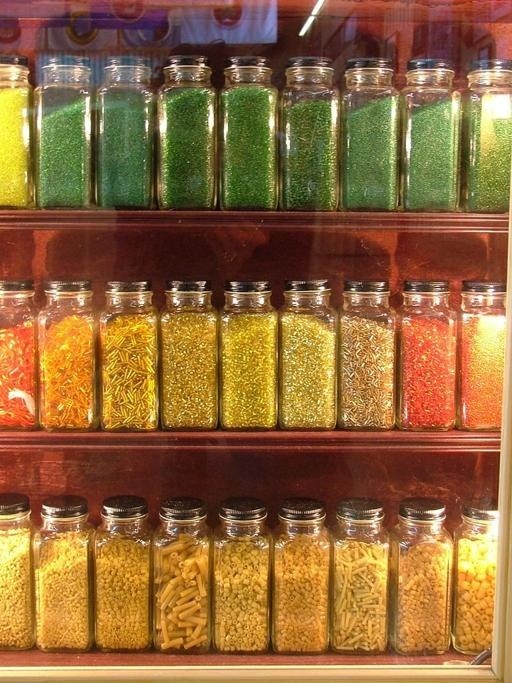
37,279,100,432
218,55,280,210
397,280,458,432
158,279,219,432
331,496,390,654
34,495,95,652
278,279,338,431
219,280,279,431
95,55,158,210
338,279,397,432
99,280,160,433
0,492,36,653
340,57,400,212
388,498,454,656
279,56,339,211
0,279,38,431
400,57,462,212
34,54,97,209
460,58,512,213
451,503,499,657
93,495,153,654
157,54,219,210
152,495,213,654
212,496,271,655
456,279,507,431
271,497,331,654
0,55,36,209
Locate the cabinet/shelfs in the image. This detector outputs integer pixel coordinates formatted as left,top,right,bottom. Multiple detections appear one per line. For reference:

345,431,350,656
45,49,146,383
0,0,512,682
